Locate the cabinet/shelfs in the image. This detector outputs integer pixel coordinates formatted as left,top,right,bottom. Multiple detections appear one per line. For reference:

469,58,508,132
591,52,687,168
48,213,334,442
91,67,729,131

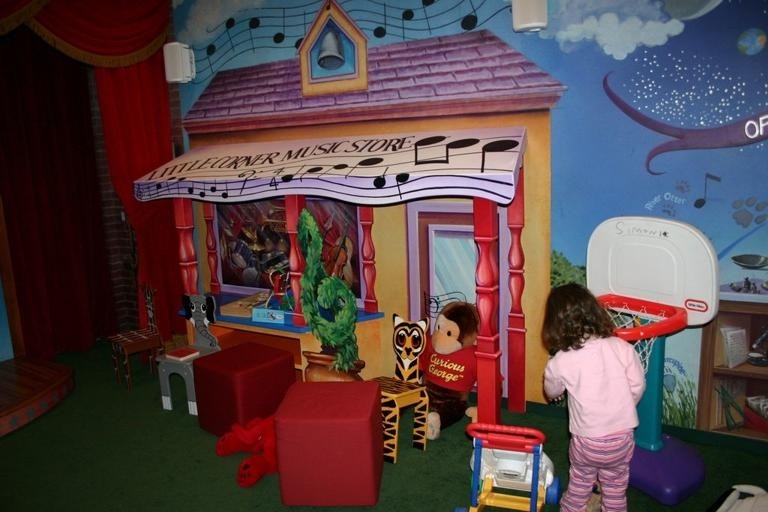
697,299,768,444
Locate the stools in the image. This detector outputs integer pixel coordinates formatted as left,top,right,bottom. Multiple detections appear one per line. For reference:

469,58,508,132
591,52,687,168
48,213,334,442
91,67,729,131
192,343,386,508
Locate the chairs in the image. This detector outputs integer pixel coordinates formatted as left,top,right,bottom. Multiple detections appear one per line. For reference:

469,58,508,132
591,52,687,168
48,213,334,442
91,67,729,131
362,313,429,465
156,291,220,418
110,286,163,391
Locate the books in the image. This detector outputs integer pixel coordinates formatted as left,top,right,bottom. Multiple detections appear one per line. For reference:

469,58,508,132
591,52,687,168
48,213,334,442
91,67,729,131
164,345,201,362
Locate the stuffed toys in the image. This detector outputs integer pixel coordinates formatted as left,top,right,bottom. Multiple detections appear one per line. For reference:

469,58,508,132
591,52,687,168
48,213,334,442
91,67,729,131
410,299,485,443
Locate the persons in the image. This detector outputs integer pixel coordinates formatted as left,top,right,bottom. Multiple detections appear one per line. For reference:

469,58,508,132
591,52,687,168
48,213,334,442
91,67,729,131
541,280,647,512
